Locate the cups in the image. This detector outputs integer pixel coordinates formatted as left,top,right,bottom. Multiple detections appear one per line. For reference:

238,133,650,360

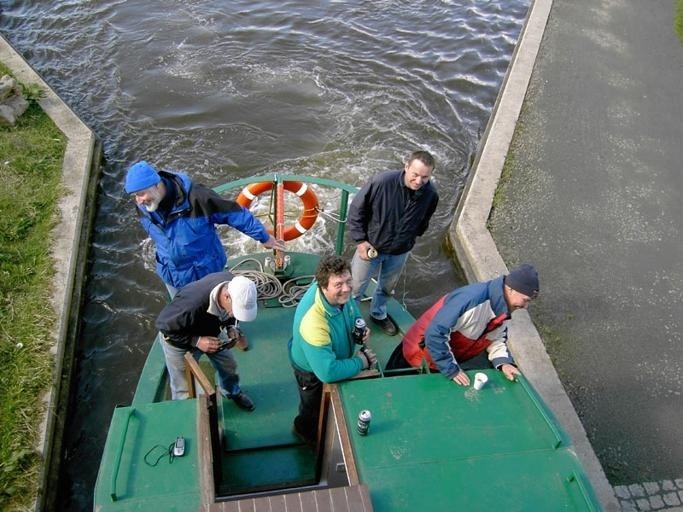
473,372,488,391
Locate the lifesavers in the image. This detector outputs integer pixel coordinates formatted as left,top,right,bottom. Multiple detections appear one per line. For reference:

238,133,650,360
235,181,319,241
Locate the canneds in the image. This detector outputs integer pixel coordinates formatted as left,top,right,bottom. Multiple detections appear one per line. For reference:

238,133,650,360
357,410,372,436
367,249,378,259
354,318,366,345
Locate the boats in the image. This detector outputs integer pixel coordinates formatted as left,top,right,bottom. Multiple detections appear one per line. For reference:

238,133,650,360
93,173,603,512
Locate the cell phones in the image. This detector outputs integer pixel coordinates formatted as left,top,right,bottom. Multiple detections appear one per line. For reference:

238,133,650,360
171,437,187,458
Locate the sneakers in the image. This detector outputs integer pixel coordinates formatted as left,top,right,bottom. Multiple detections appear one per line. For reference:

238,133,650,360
291,425,317,446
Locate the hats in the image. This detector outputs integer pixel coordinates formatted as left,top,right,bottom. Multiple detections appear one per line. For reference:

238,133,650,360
227,274,259,322
123,160,161,196
503,262,540,300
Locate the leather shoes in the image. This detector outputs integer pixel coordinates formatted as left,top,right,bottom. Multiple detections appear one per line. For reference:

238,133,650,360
226,324,248,351
229,391,256,412
369,312,396,336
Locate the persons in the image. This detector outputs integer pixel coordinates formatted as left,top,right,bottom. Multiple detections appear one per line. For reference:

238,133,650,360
123,160,287,351
288,255,378,446
384,263,539,385
154,273,258,412
348,151,438,338
